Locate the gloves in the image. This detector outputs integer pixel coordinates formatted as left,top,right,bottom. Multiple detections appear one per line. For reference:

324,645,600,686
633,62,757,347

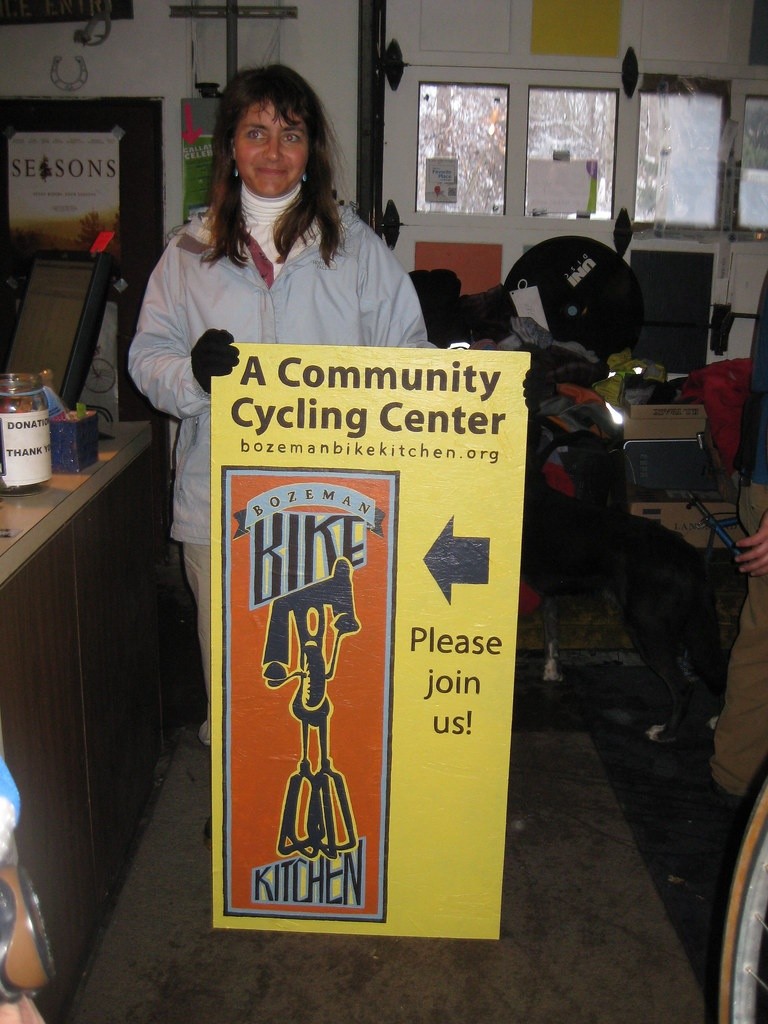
190,328,240,394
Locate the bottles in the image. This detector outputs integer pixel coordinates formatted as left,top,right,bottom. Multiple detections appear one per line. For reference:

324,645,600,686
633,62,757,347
0,373,52,498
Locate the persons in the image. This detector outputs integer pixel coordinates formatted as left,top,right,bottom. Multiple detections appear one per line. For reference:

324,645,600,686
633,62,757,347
710,273,768,809
128,66,546,850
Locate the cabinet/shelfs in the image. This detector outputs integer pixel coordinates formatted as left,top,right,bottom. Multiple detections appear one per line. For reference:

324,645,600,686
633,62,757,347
0,419,165,1024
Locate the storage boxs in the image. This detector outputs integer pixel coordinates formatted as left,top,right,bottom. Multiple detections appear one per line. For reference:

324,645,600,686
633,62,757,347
49,410,100,471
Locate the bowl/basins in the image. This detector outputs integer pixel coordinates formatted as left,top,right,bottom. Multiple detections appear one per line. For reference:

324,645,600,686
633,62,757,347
47,408,100,475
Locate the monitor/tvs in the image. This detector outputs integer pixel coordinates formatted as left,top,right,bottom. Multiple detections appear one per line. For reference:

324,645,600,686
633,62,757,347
0,248,116,413
